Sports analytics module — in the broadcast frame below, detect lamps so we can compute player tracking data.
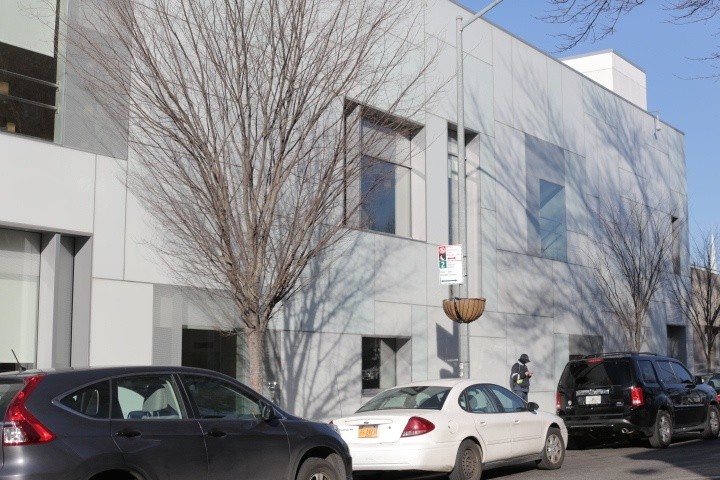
[650,111,660,130]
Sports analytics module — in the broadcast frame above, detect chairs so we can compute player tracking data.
[463,395,485,413]
[418,397,438,408]
[77,390,99,416]
[143,384,179,419]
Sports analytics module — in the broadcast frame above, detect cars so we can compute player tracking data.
[698,373,720,401]
[1,365,353,480]
[328,377,569,480]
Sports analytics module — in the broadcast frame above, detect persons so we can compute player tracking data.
[512,354,533,401]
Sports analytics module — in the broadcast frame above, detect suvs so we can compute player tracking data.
[555,349,720,448]
